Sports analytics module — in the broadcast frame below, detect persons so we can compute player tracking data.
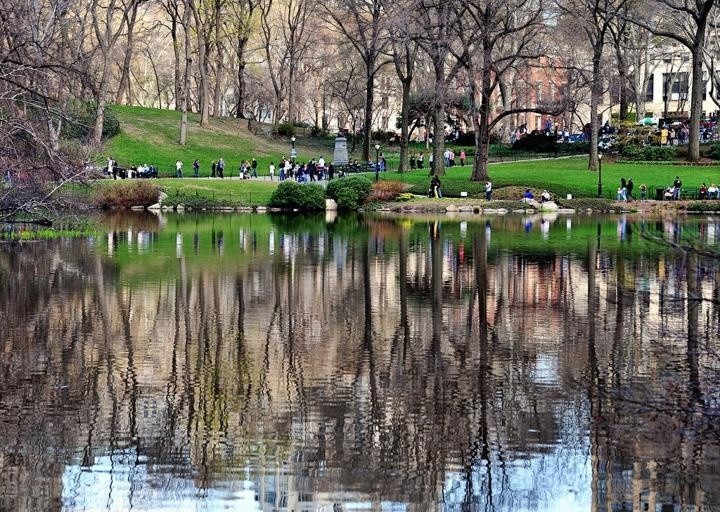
[524,189,535,198]
[698,181,720,199]
[444,147,457,167]
[239,157,257,180]
[5,221,30,234]
[192,159,199,178]
[210,157,225,178]
[672,176,682,201]
[540,189,551,202]
[347,153,387,173]
[418,152,424,169]
[4,160,29,184]
[617,176,637,201]
[279,156,334,182]
[175,160,183,178]
[640,184,646,200]
[107,156,157,181]
[427,175,443,199]
[615,213,720,245]
[430,153,433,168]
[597,113,719,147]
[460,149,465,166]
[485,179,493,201]
[269,162,275,180]
[409,153,416,170]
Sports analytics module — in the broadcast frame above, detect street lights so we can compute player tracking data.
[597,152,603,196]
[291,135,296,169]
[374,144,381,180]
